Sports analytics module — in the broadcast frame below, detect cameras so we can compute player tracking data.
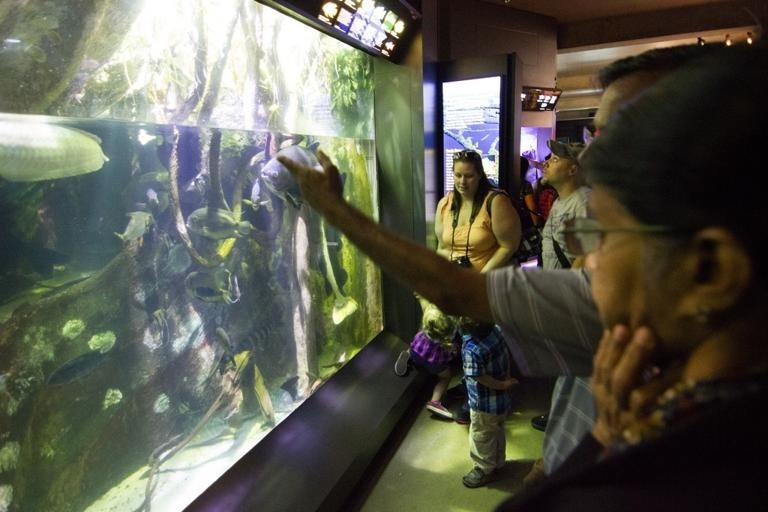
[451,255,472,269]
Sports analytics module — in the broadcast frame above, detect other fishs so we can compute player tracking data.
[1,110,364,431]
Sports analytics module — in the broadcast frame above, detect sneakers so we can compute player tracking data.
[461,462,508,489]
[425,399,454,420]
[393,349,411,376]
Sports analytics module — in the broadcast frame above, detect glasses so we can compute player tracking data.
[451,150,479,162]
[555,215,673,257]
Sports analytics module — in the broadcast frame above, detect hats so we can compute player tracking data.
[545,139,585,162]
